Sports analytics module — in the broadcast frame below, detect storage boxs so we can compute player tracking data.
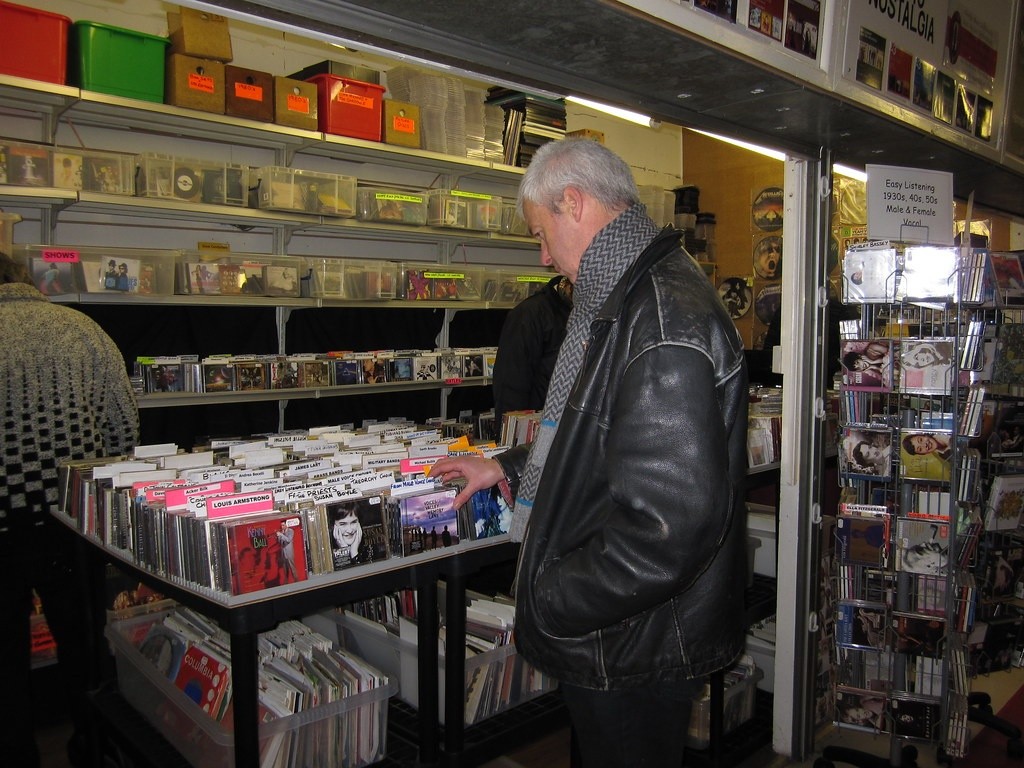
[223,64,275,124]
[0,138,558,307]
[638,181,717,270]
[686,666,765,753]
[73,20,172,103]
[272,74,319,132]
[302,581,566,730]
[164,5,234,61]
[381,97,423,149]
[166,53,225,114]
[105,597,401,767]
[284,60,387,142]
[0,1,72,85]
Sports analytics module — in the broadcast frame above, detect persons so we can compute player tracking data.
[275,520,300,584]
[721,281,749,318]
[105,259,130,291]
[0,253,141,767]
[832,248,1020,741]
[758,238,784,277]
[491,273,585,447]
[329,502,367,567]
[464,356,484,376]
[363,360,385,384]
[423,139,754,768]
[191,265,207,294]
[411,525,452,550]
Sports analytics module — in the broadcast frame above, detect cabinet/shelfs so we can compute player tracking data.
[0,70,563,410]
[45,503,578,767]
[826,222,1022,743]
